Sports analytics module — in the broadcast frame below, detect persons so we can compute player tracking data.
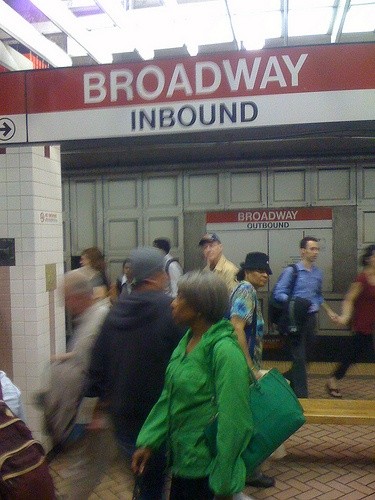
[0,233,375,500]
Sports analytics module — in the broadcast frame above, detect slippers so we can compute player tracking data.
[326,383,342,398]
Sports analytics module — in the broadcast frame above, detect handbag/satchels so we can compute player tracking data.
[268,293,313,336]
[204,367,307,482]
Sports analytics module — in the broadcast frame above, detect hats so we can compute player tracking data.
[128,246,166,279]
[199,233,220,246]
[240,252,272,275]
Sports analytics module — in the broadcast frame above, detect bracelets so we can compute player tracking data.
[329,314,336,320]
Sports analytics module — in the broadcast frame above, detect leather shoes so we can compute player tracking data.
[245,476,275,488]
